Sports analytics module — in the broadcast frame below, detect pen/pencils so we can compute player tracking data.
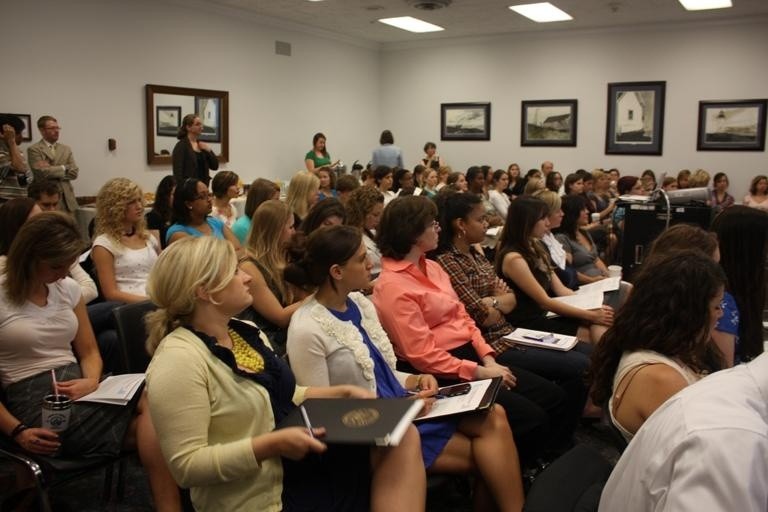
[51,369,59,402]
[522,335,544,342]
[404,390,444,399]
[299,405,315,438]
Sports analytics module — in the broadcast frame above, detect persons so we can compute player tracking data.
[369,128,402,177]
[0,162,768,512]
[419,141,445,171]
[171,113,220,191]
[0,111,35,205]
[303,133,341,177]
[26,114,79,210]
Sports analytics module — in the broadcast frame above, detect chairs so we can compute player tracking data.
[524,445,612,511]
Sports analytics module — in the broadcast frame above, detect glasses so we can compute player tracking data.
[189,191,213,201]
[42,126,60,131]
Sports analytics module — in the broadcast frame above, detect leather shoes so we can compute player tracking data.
[521,457,554,491]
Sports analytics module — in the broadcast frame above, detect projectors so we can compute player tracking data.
[663,187,712,207]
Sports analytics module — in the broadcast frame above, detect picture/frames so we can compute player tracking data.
[440,101,490,141]
[605,81,665,155]
[157,106,181,136]
[10,114,32,141]
[194,95,220,142]
[697,99,767,151]
[520,99,577,147]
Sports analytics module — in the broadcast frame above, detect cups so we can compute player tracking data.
[608,264,623,279]
[41,395,72,434]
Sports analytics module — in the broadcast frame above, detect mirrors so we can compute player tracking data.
[146,84,228,164]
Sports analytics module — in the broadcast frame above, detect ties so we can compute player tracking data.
[50,145,55,155]
